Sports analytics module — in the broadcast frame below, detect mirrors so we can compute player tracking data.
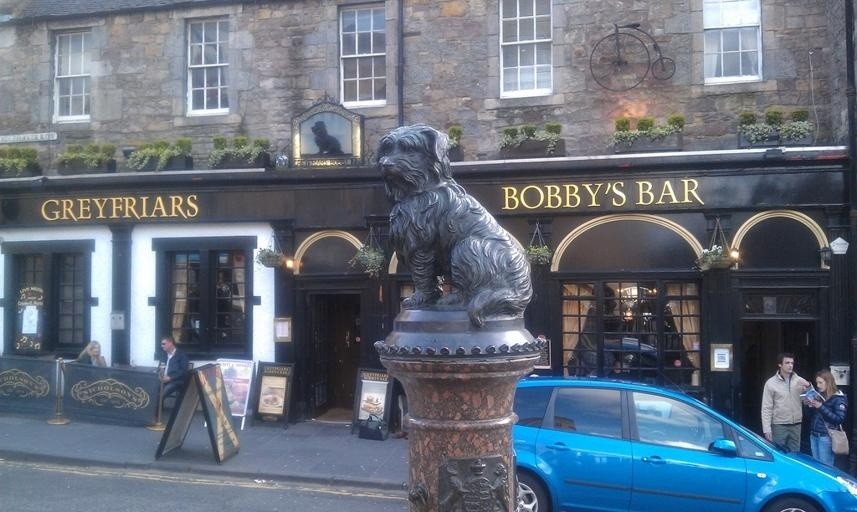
[291,90,364,169]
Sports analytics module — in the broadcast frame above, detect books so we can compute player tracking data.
[800,388,825,409]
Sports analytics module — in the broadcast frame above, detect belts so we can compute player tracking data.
[811,431,826,437]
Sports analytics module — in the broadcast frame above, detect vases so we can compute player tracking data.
[706,257,731,270]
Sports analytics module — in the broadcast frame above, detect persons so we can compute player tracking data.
[763,350,813,453]
[804,368,849,467]
[160,334,188,399]
[78,340,101,365]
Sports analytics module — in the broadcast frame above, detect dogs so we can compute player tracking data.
[377,124,534,329]
[311,120,344,155]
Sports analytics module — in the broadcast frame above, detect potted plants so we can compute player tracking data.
[208,135,270,169]
[0,144,42,177]
[122,137,193,172]
[445,126,464,162]
[524,245,553,265]
[256,246,286,268]
[736,108,814,149]
[496,123,566,159]
[606,112,686,153]
[53,143,116,175]
[348,246,386,276]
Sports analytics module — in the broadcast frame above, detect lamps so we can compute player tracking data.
[285,257,294,270]
[730,244,739,260]
[818,245,832,261]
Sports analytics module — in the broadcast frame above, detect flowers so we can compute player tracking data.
[691,245,738,261]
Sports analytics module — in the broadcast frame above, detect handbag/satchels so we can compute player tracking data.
[827,428,850,456]
[358,414,389,441]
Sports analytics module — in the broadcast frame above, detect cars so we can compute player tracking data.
[511,377,857,512]
[600,339,675,379]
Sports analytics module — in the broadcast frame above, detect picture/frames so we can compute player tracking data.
[710,343,735,372]
[275,317,293,343]
